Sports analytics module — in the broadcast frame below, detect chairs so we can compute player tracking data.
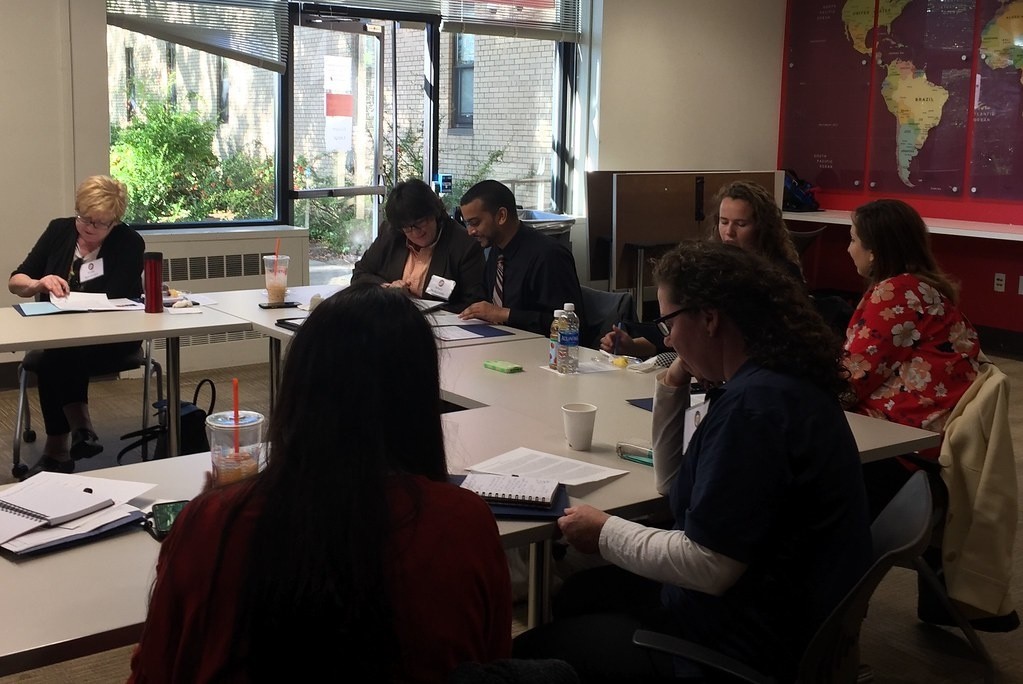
[10,285,1009,684]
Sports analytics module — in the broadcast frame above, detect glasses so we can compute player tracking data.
[398,214,430,233]
[77,214,116,229]
[653,301,698,337]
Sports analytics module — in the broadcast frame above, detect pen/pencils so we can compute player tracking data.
[62,285,65,290]
[613,323,621,355]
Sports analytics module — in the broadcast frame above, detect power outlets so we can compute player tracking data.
[994,273,1005,292]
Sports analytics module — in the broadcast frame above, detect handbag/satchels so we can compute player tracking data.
[152,396,213,461]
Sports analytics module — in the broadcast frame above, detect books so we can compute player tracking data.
[458,472,560,510]
[0,479,115,546]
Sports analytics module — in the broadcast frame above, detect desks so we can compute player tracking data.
[0,406,668,684]
[439,337,940,466]
[188,286,546,426]
[0,305,254,479]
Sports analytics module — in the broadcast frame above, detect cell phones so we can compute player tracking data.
[484,360,523,373]
[258,302,297,309]
[152,500,192,537]
[690,383,706,394]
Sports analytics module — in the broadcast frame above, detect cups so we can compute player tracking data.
[205,411,264,487]
[263,254,290,303]
[562,403,598,451]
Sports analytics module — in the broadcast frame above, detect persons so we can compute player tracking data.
[129,280,515,684]
[601,182,805,363]
[507,236,870,684]
[834,198,982,501]
[456,180,584,345]
[8,175,146,475]
[349,178,488,312]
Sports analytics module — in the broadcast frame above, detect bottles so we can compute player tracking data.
[549,310,569,370]
[558,303,579,374]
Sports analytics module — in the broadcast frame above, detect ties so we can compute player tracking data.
[492,254,504,325]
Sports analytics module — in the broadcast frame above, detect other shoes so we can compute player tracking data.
[69,426,105,461]
[19,453,76,483]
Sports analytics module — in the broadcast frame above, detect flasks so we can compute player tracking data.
[145,251,163,313]
